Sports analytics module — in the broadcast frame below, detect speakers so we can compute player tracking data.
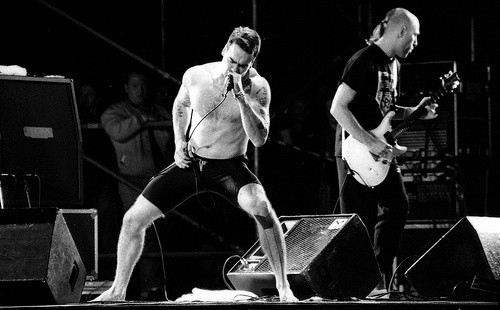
[0,208,87,306]
[404,217,500,299]
[226,213,382,301]
[0,75,87,206]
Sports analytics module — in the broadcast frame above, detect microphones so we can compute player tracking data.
[226,75,233,91]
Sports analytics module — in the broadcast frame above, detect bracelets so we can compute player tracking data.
[234,90,246,100]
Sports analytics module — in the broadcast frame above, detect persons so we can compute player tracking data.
[283,96,340,214]
[87,26,299,303]
[330,7,439,300]
[78,72,171,213]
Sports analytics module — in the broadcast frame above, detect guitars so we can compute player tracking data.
[340,70,463,189]
